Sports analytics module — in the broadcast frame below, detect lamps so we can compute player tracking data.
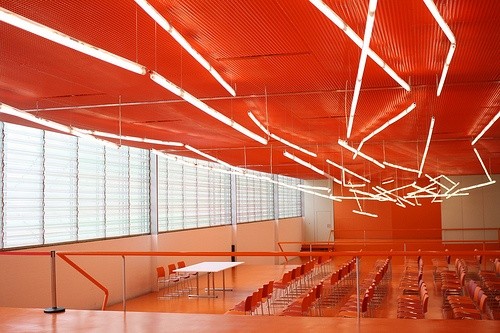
[1,1,499,218]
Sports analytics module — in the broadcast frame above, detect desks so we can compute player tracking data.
[173,261,244,298]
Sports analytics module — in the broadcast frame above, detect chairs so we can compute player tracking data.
[156,266,171,298]
[177,260,192,293]
[225,253,499,320]
[167,264,181,296]
